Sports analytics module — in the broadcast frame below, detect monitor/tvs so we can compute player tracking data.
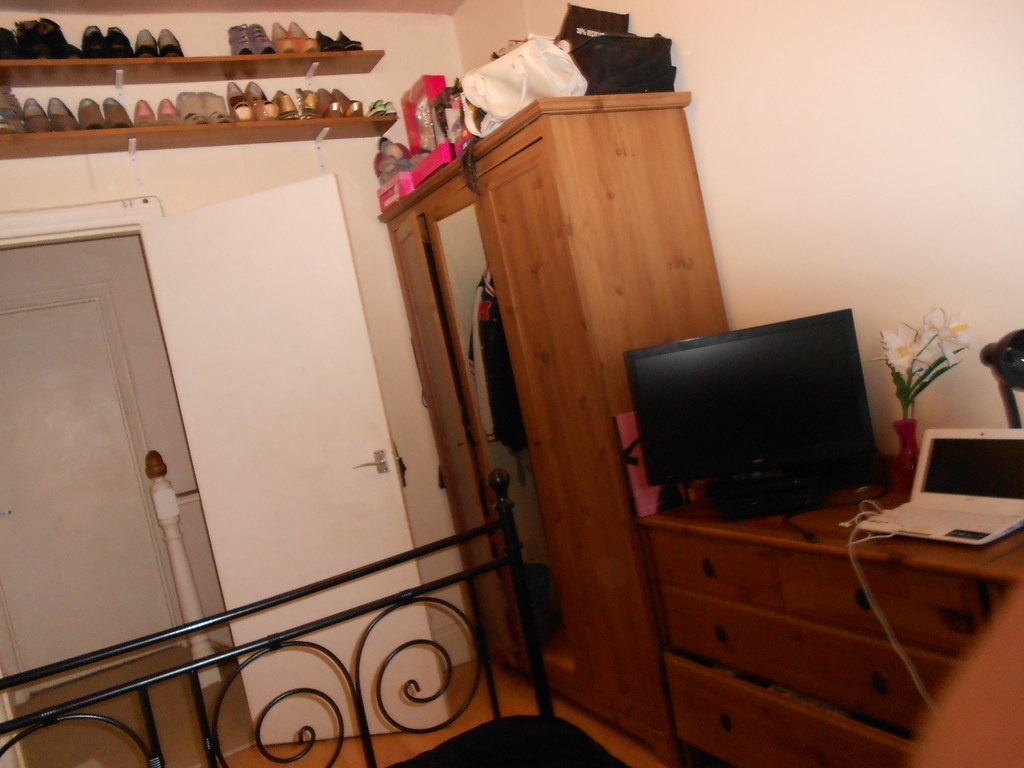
[625,306,876,486]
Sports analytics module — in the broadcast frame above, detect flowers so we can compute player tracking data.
[870,305,970,421]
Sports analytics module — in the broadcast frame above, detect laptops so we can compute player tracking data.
[856,428,1024,546]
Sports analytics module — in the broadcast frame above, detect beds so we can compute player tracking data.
[0,469,631,768]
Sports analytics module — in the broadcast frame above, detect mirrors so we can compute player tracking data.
[435,203,574,669]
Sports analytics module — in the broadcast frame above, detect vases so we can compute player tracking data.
[893,419,921,488]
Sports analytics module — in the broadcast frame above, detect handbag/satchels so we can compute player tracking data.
[461,32,677,136]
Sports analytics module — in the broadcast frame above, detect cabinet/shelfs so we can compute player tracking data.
[379,93,730,768]
[0,50,400,161]
[635,454,1024,768]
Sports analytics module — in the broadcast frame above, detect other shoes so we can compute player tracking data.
[0,83,398,134]
[1,17,364,61]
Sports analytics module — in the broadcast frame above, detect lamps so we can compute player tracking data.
[979,329,1024,429]
